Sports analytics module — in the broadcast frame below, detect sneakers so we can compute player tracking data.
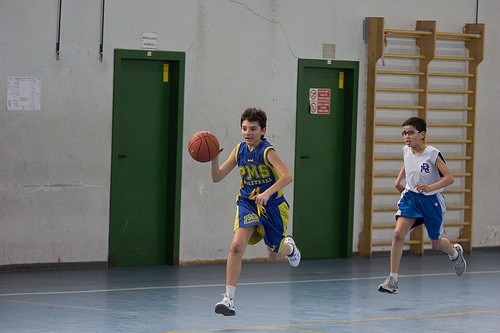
[214,293,236,316]
[377,275,399,294]
[284,235,302,268]
[449,243,466,277]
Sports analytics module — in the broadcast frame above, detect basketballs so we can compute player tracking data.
[187,131,219,163]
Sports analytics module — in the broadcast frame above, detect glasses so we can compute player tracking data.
[401,130,423,137]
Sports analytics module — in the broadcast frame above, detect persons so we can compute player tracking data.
[212,109,301,316]
[377,116,467,294]
